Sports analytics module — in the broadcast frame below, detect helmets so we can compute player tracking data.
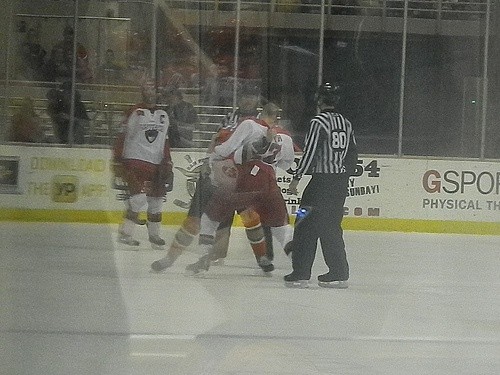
[316,82,343,99]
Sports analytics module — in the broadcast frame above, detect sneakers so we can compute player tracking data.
[117,230,140,251]
[257,254,274,277]
[149,234,166,251]
[151,256,173,273]
[318,271,349,288]
[183,255,211,278]
[284,269,311,288]
[208,248,224,267]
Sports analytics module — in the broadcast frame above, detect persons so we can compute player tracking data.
[150,84,267,272]
[8,26,199,150]
[283,80,359,288]
[186,101,296,271]
[110,77,175,247]
